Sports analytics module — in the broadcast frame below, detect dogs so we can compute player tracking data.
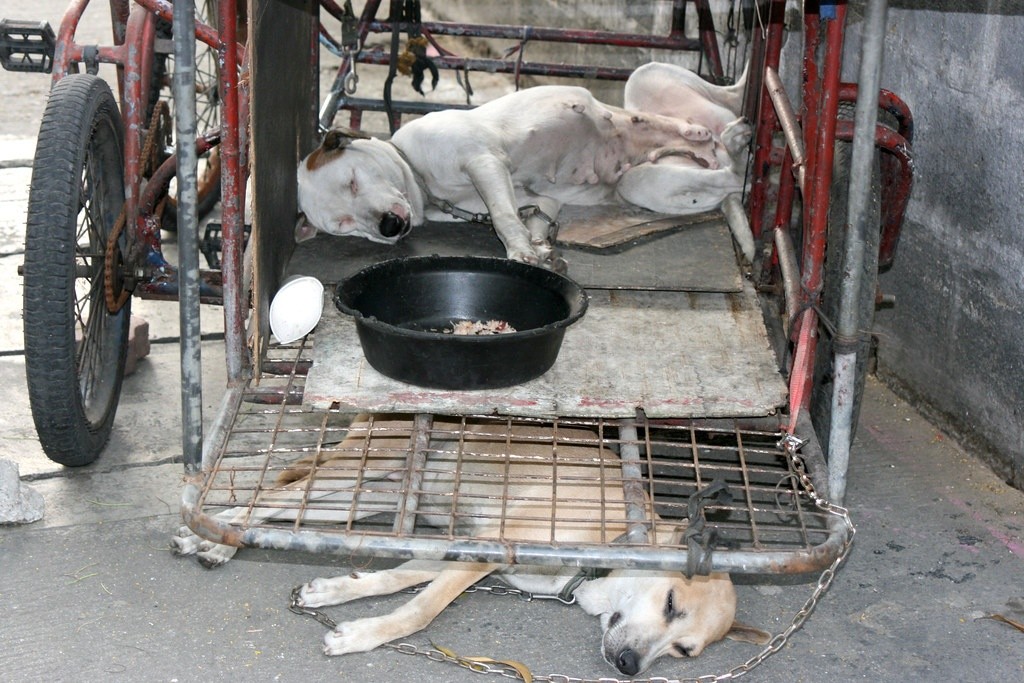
[295,58,759,265]
[169,414,773,677]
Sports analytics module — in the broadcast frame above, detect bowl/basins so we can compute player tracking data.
[333,254,590,391]
